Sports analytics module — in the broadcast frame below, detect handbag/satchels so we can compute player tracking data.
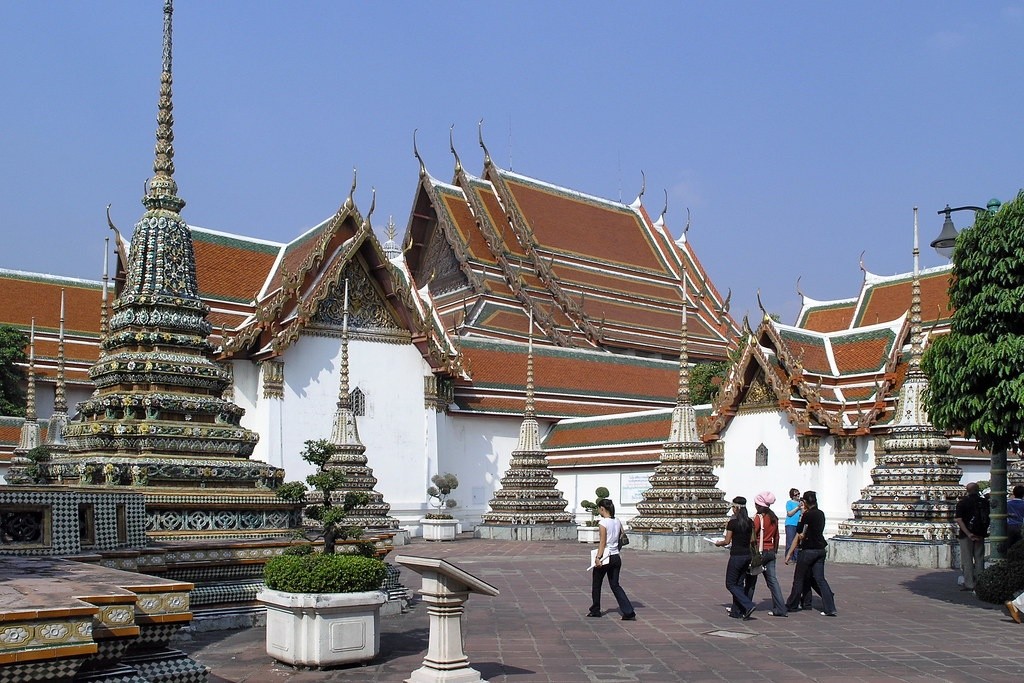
[618,528,629,550]
[969,517,990,538]
[749,554,765,576]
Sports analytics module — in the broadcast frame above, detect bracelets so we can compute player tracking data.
[596,556,601,559]
[798,505,801,507]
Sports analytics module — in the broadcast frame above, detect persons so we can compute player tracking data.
[715,495,757,620]
[1007,485,1024,528]
[956,482,990,592]
[1005,591,1024,624]
[784,492,838,615]
[783,489,802,564]
[725,491,788,617]
[586,498,636,620]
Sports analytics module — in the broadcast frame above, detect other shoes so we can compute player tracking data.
[768,611,783,616]
[821,610,837,616]
[799,603,813,610]
[620,612,636,620]
[785,604,797,612]
[959,584,974,591]
[725,607,732,612]
[586,611,601,617]
[743,606,757,621]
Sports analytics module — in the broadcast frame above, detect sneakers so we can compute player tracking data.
[1005,601,1021,624]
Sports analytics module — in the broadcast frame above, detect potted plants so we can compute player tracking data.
[577,520,601,543]
[256,554,390,667]
[419,512,458,541]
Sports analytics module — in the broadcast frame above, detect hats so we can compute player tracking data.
[726,500,747,508]
[755,492,776,507]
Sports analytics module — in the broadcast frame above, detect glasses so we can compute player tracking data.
[794,493,800,496]
